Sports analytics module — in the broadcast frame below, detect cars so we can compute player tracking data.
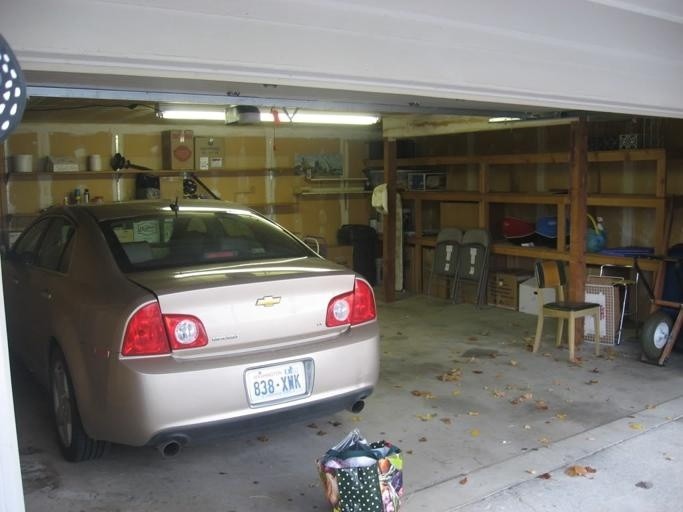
[2,199,381,464]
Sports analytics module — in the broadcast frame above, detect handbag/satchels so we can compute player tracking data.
[316,430,402,512]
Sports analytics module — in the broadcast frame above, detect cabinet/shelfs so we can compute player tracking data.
[1,165,303,218]
[362,148,668,330]
[296,177,374,196]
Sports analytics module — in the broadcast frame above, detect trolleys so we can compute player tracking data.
[630,194,682,370]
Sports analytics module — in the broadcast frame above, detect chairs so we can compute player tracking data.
[120,240,156,265]
[530,259,602,363]
[452,226,490,309]
[599,263,640,345]
[426,226,462,302]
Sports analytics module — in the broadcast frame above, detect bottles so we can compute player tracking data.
[61,188,89,205]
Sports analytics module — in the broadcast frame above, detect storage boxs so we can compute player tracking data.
[194,135,225,168]
[585,274,622,338]
[408,172,448,194]
[161,128,193,168]
[324,245,353,274]
[367,168,418,193]
[463,171,514,194]
[487,268,534,311]
[160,176,185,201]
[440,203,480,232]
[422,248,477,303]
[519,277,556,316]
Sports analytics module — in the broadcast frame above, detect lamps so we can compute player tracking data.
[154,102,383,125]
